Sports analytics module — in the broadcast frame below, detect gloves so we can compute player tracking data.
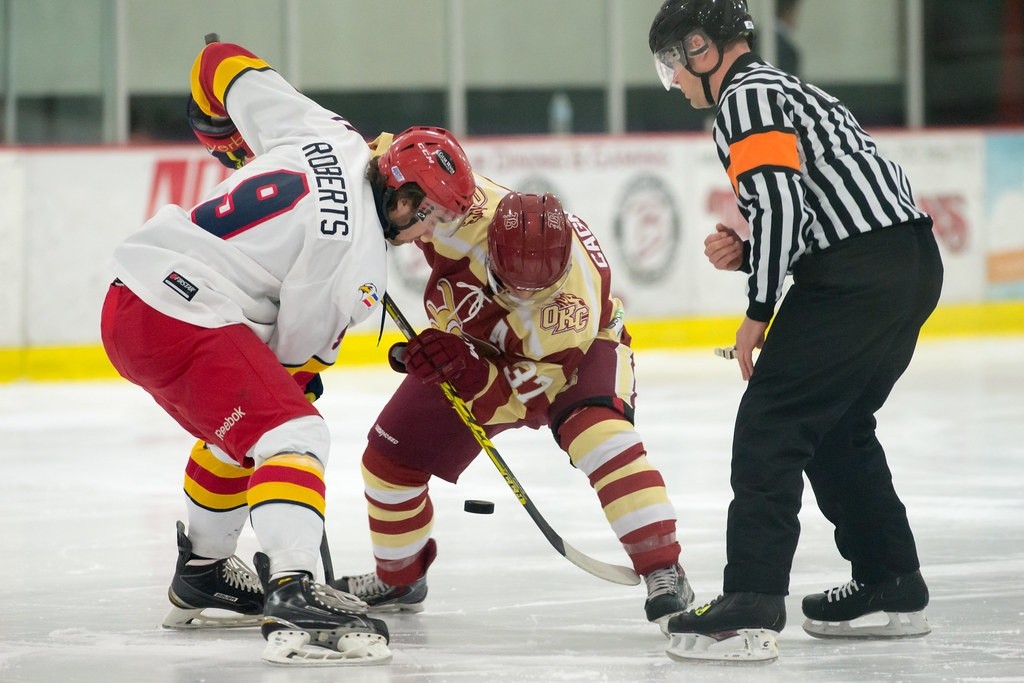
[402,328,490,403]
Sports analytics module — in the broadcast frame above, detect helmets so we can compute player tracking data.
[379,127,477,215]
[649,0,756,76]
[486,189,572,294]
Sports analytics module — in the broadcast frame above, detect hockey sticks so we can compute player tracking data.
[382,293,642,586]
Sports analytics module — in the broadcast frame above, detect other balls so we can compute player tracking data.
[462,498,495,515]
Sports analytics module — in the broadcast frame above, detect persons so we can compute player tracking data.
[335,132,694,633]
[102,44,475,666]
[648,1,944,660]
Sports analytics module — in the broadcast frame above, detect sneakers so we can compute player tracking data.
[801,572,930,641]
[662,594,786,660]
[253,551,394,667]
[326,537,437,614]
[163,519,265,631]
[642,564,696,624]
